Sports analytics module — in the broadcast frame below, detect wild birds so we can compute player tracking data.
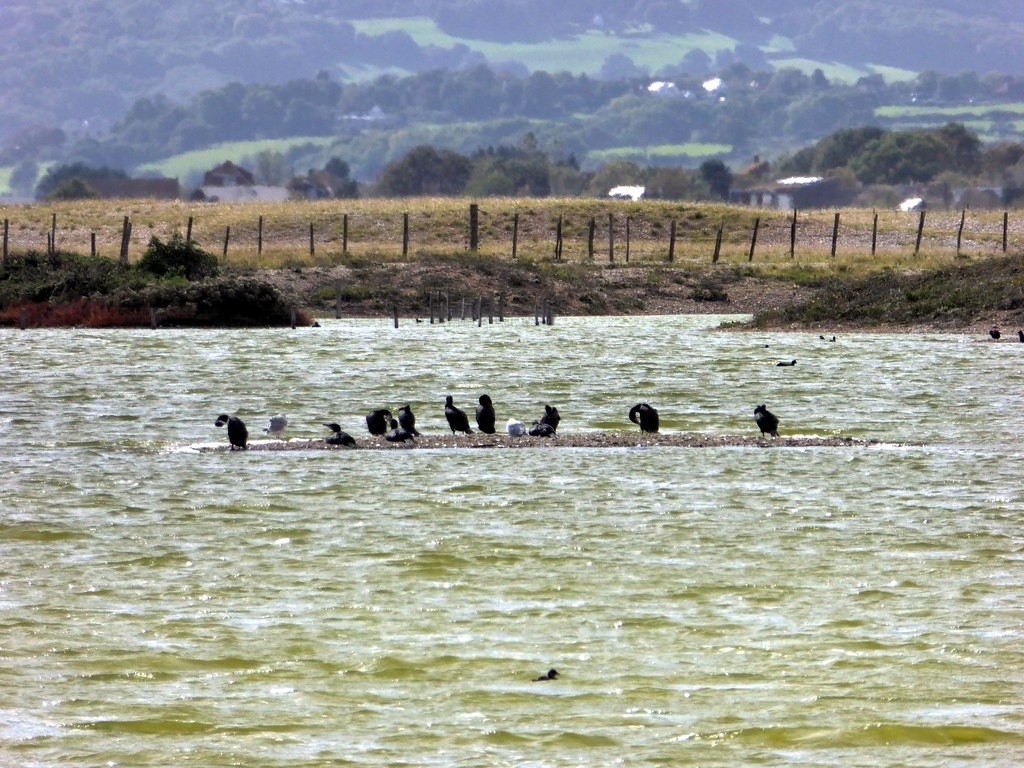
[531,667,562,683]
[775,359,797,367]
[214,412,290,450]
[753,402,783,438]
[629,403,661,436]
[988,329,1024,342]
[415,316,424,323]
[322,405,425,451]
[444,393,561,436]
[764,345,769,348]
[312,321,321,327]
[819,334,837,342]
